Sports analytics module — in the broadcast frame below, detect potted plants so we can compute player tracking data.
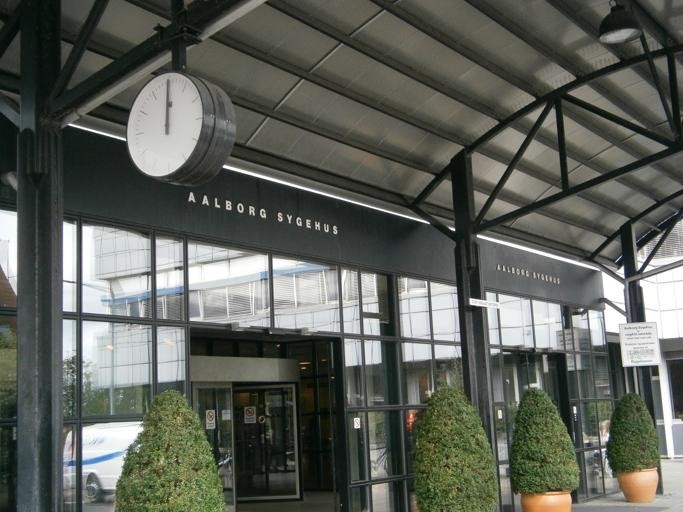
[604,391,660,503]
[507,386,582,512]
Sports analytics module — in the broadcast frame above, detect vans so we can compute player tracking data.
[61,420,147,503]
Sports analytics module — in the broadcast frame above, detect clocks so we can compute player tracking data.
[125,69,237,187]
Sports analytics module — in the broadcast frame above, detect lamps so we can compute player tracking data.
[598,4,643,46]
